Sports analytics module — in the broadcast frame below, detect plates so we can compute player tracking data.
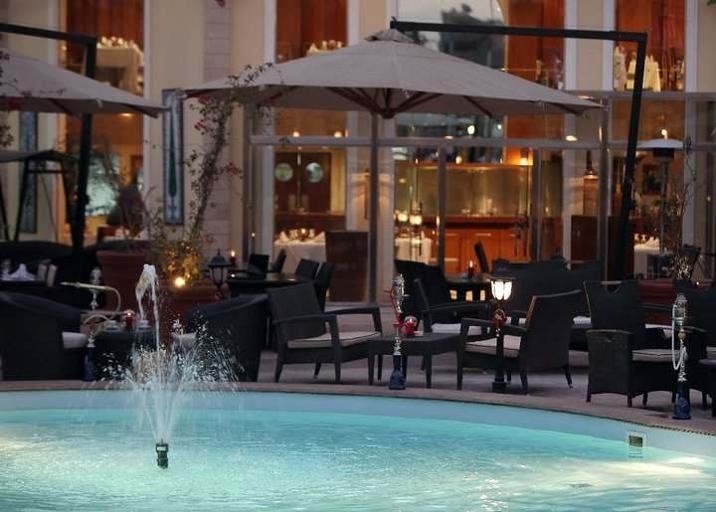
[140,326,153,329]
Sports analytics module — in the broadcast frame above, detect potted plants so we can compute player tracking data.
[88,133,169,310]
[636,136,712,325]
[153,61,286,306]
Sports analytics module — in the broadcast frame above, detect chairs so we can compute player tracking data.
[0,244,716,418]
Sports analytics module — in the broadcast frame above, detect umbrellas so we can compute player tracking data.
[182,20,649,276]
[0,23,169,249]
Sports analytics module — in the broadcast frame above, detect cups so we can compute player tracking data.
[140,320,149,325]
[413,330,424,336]
[505,316,527,324]
